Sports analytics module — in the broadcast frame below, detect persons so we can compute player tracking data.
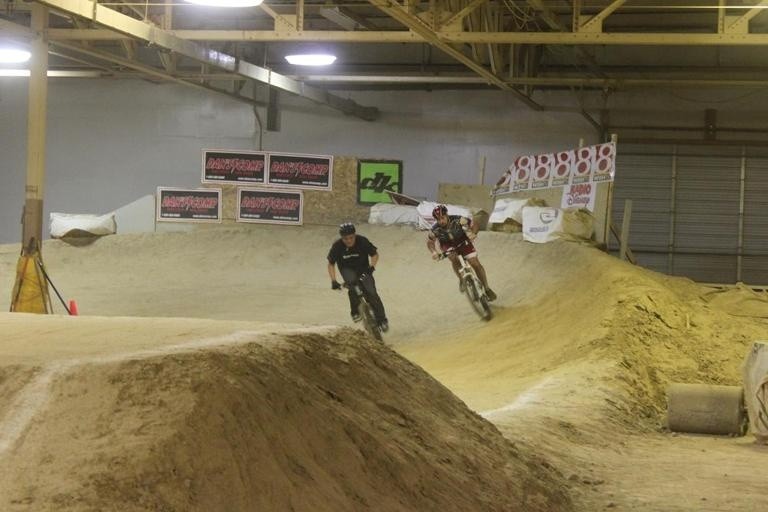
[428,203,497,301]
[327,223,388,332]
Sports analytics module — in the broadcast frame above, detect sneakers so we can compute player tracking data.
[379,321,389,333]
[458,280,468,293]
[486,288,497,300]
[351,311,362,324]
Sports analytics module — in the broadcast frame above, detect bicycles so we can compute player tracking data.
[435,231,494,324]
[336,268,383,344]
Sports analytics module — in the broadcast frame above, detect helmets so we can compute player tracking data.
[337,222,357,237]
[431,204,448,219]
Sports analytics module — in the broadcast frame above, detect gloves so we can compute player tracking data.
[367,266,376,276]
[331,279,342,291]
